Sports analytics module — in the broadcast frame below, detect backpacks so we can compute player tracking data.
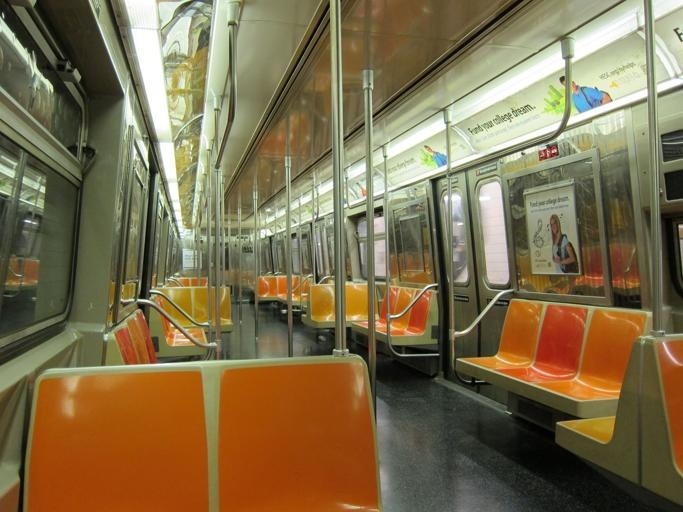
[561,264,577,273]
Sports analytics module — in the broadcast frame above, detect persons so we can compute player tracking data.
[549,215,578,274]
[424,146,448,168]
[559,75,612,113]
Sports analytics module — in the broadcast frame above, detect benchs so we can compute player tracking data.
[518,243,641,296]
[301,283,351,344]
[107,309,158,364]
[554,335,639,506]
[148,287,208,358]
[4,258,38,293]
[165,276,210,287]
[389,252,432,283]
[353,285,441,376]
[23,353,382,512]
[639,331,681,509]
[192,286,231,339]
[456,298,654,425]
[259,275,313,321]
